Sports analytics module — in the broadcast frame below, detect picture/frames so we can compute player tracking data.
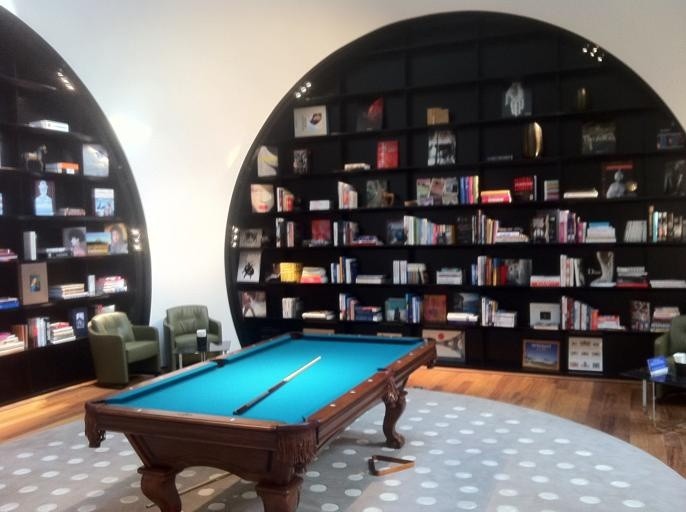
[289,101,331,140]
[420,328,470,365]
[15,259,50,307]
[521,337,560,372]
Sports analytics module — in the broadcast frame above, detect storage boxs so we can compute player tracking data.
[27,119,69,133]
[45,160,78,176]
[309,200,331,210]
[435,269,463,285]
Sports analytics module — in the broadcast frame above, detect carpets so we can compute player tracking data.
[0,387,686,512]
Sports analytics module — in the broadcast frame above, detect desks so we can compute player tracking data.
[84,329,437,512]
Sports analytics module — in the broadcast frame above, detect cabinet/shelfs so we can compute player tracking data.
[224,9,685,383]
[0,8,152,401]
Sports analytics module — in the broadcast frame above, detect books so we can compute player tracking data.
[237,80,686,376]
[0,117,132,358]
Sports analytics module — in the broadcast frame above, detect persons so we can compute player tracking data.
[107,226,127,255]
[241,292,255,319]
[34,181,53,216]
[249,183,274,213]
[67,229,85,257]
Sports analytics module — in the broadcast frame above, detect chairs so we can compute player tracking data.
[655,317,685,402]
[164,304,225,369]
[86,311,164,384]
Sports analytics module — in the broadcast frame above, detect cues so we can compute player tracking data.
[232,355,322,413]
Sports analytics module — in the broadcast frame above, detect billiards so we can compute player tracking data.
[385,391,399,403]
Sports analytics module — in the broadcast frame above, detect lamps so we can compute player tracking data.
[582,43,606,64]
[295,79,312,100]
